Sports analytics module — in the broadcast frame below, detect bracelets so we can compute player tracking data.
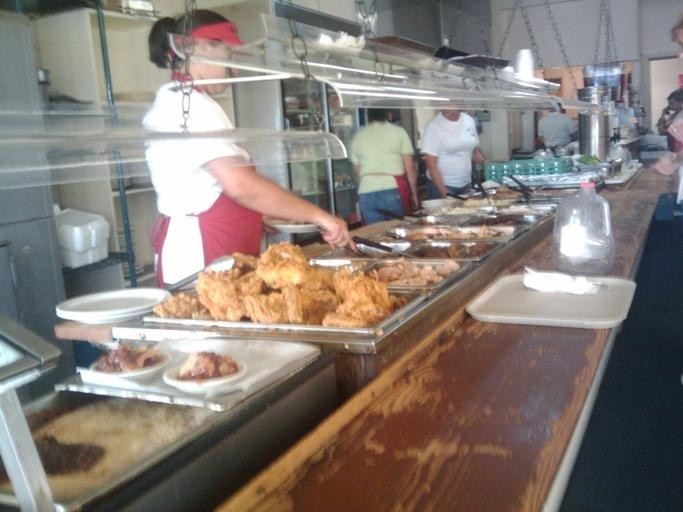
[481,158,487,164]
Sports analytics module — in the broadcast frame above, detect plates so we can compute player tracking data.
[54,285,173,326]
[420,198,447,211]
[263,215,323,234]
[88,355,248,393]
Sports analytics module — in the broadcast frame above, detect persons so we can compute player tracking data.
[658,88,683,217]
[348,108,420,225]
[420,108,488,200]
[536,103,577,155]
[142,9,358,293]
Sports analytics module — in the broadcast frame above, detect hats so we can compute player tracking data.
[174,22,247,48]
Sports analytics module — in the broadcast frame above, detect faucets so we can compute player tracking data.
[608,123,624,143]
